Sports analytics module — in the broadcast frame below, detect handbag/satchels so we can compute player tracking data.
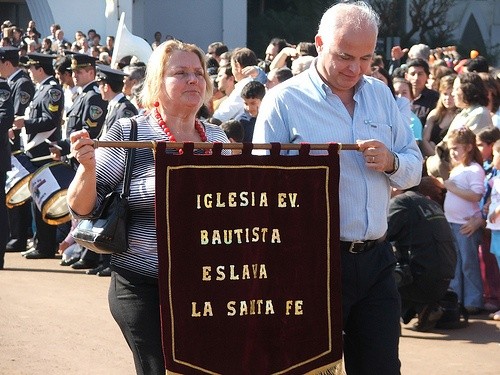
[73,192,131,254]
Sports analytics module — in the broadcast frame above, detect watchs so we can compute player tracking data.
[384,152,399,175]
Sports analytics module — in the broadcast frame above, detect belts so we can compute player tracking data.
[340,231,388,254]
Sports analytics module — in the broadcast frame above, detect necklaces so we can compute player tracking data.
[154,107,212,156]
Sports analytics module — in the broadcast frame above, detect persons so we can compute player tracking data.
[66,38,232,375]
[0,19,500,331]
[251,0,423,375]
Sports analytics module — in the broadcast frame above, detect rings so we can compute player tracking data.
[370,156,375,163]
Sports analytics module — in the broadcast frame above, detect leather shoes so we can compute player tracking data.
[60,258,80,266]
[86,264,112,276]
[21,247,56,259]
[72,259,94,269]
[5,239,26,252]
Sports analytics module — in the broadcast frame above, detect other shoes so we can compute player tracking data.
[412,301,445,332]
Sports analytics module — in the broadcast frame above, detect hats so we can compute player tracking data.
[98,67,129,82]
[0,46,21,59]
[66,53,99,69]
[24,53,58,68]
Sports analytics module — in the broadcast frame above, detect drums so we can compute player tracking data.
[5,151,39,211]
[29,160,78,226]
